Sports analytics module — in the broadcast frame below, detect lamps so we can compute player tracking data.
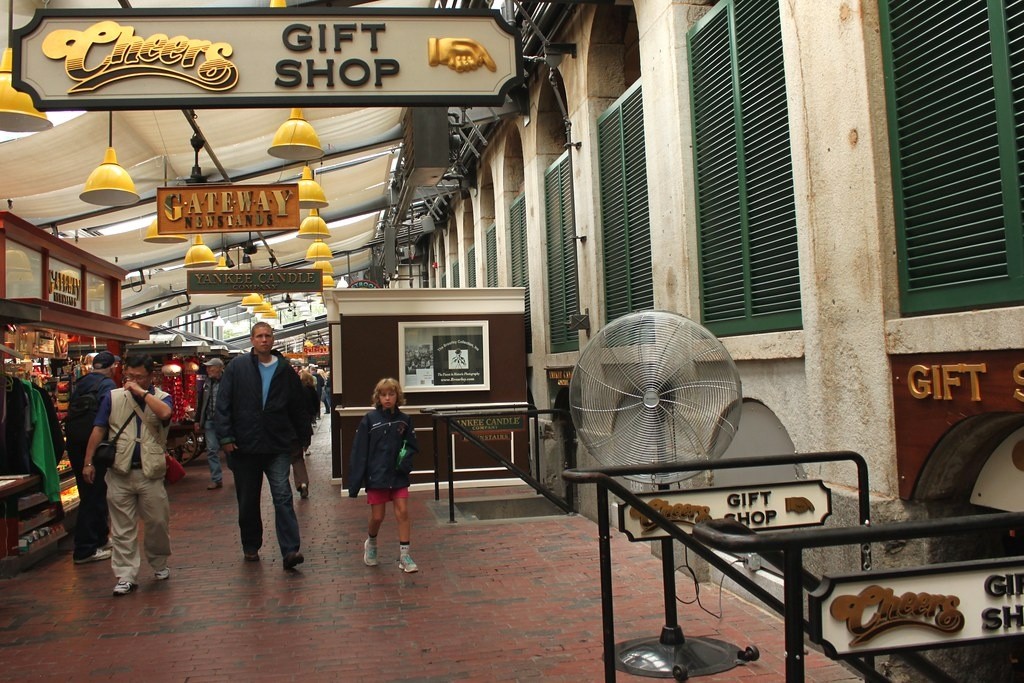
[227,291,279,320]
[182,233,218,267]
[312,262,334,276]
[78,111,142,205]
[266,108,325,162]
[0,33,53,135]
[305,237,335,262]
[143,153,188,244]
[316,275,336,304]
[216,256,229,271]
[298,208,329,238]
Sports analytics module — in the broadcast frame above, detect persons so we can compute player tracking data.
[83,353,172,596]
[214,322,313,571]
[299,371,317,456]
[292,364,332,420]
[65,352,121,564]
[347,378,421,574]
[193,357,225,491]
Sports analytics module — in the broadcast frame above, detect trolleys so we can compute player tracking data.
[166,425,207,467]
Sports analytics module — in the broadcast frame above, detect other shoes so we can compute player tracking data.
[300,483,309,497]
[207,482,223,489]
[284,551,304,569]
[244,551,259,561]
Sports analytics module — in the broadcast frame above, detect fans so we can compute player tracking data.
[281,294,303,319]
[571,311,742,677]
[225,231,262,270]
[267,254,278,271]
[134,127,250,185]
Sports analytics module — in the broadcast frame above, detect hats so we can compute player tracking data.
[92,351,121,369]
[293,361,303,366]
[204,358,225,366]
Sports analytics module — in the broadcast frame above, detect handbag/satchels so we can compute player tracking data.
[94,442,116,467]
[164,452,187,486]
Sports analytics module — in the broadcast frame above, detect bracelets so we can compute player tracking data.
[141,390,150,399]
[84,463,93,466]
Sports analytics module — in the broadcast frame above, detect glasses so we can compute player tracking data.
[125,373,150,381]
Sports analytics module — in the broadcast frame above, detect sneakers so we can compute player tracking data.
[73,549,112,564]
[399,554,419,573]
[113,582,134,594]
[154,566,170,579]
[98,537,113,548]
[364,540,379,567]
